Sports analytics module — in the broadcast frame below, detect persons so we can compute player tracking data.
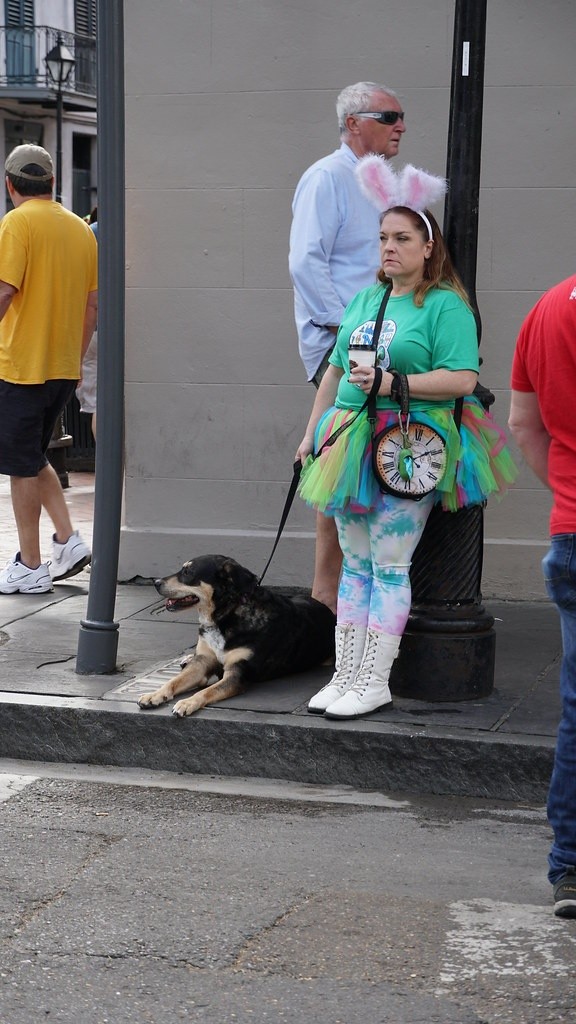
[508,274,576,919]
[0,144,97,594]
[292,205,519,719]
[285,77,407,617]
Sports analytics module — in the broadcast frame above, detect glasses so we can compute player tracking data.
[356,111,404,125]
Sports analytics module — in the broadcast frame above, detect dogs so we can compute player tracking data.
[137,555,337,717]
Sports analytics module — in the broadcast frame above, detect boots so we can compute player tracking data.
[324,626,402,720]
[307,625,367,712]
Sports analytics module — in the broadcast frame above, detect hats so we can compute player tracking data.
[5,144,53,181]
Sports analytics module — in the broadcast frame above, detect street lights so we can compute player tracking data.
[44,31,79,204]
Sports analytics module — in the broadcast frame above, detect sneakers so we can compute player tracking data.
[553,865,576,915]
[49,530,91,581]
[0,551,54,594]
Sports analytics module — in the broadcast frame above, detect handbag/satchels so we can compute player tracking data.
[372,420,448,501]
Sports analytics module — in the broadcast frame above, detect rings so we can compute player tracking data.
[363,376,367,383]
